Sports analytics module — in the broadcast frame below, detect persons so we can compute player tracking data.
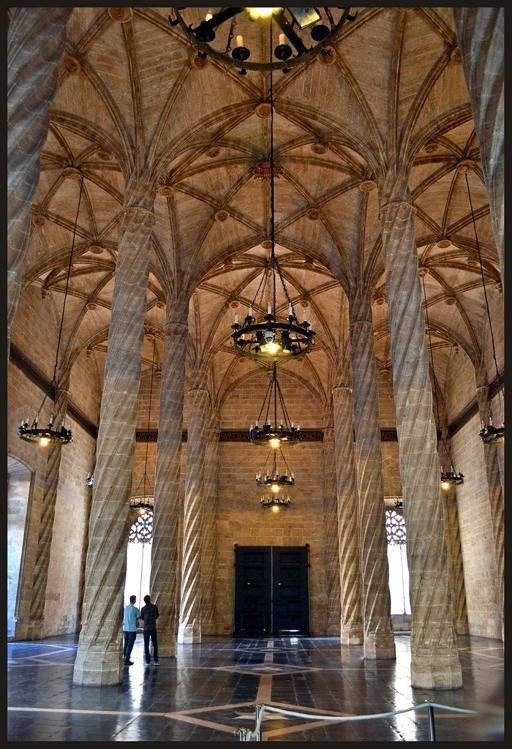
[122,595,142,665]
[140,595,160,666]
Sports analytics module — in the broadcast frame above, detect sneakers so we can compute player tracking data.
[124,660,134,665]
[145,660,151,665]
[153,661,161,665]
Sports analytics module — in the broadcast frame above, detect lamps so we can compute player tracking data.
[131,343,160,515]
[85,472,93,488]
[19,174,91,448]
[421,273,464,489]
[394,490,403,510]
[255,448,295,514]
[229,26,315,363]
[462,170,505,448]
[167,1,360,78]
[249,364,303,450]
